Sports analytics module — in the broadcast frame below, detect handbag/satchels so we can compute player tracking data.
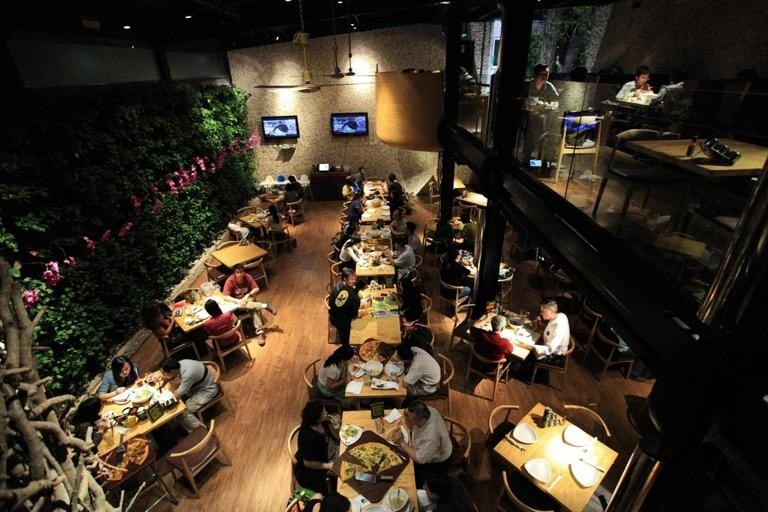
[293,487,316,502]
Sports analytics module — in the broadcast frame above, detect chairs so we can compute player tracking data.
[204,257,227,286]
[176,361,236,427]
[166,419,231,503]
[171,287,252,330]
[216,240,237,249]
[204,320,252,375]
[593,128,664,221]
[534,109,603,183]
[158,334,200,360]
[282,173,660,512]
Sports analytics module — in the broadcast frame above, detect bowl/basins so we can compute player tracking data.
[508,317,525,329]
[640,94,654,103]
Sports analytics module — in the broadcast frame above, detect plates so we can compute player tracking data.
[382,258,391,265]
[512,423,538,443]
[363,205,390,219]
[111,387,174,405]
[563,424,589,446]
[387,487,409,511]
[338,424,364,445]
[359,504,385,512]
[185,306,200,315]
[385,425,409,447]
[569,462,600,488]
[106,418,116,428]
[524,456,552,484]
[347,359,405,378]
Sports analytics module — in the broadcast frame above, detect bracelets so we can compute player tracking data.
[400,441,406,449]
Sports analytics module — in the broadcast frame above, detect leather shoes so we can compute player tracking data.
[257,333,264,345]
[265,303,275,315]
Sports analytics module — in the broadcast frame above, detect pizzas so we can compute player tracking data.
[103,438,149,482]
[358,340,387,363]
[340,441,404,485]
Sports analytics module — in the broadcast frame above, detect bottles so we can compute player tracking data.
[687,134,699,157]
[541,407,552,427]
[495,305,506,316]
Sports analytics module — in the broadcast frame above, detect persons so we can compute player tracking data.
[203,299,241,365]
[431,211,570,386]
[390,399,456,491]
[261,205,284,248]
[282,175,305,222]
[141,302,200,360]
[150,356,220,435]
[645,71,689,111]
[273,121,288,136]
[222,264,277,346]
[314,344,364,409]
[57,397,108,453]
[97,354,142,403]
[342,117,358,133]
[521,63,561,160]
[613,65,662,125]
[300,489,354,511]
[390,344,442,395]
[330,166,434,355]
[291,399,340,491]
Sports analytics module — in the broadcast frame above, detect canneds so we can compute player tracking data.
[540,407,557,428]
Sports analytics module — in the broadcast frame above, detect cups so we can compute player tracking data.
[550,101,558,110]
[116,406,148,428]
[103,433,113,445]
[200,283,210,296]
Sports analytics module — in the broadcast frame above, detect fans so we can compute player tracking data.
[253,27,357,95]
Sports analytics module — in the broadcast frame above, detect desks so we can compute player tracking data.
[311,159,348,201]
[167,286,240,361]
[86,369,187,512]
[627,136,768,233]
[210,241,266,275]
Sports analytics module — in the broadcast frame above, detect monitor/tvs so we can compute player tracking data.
[319,163,330,171]
[262,115,300,139]
[331,112,369,136]
